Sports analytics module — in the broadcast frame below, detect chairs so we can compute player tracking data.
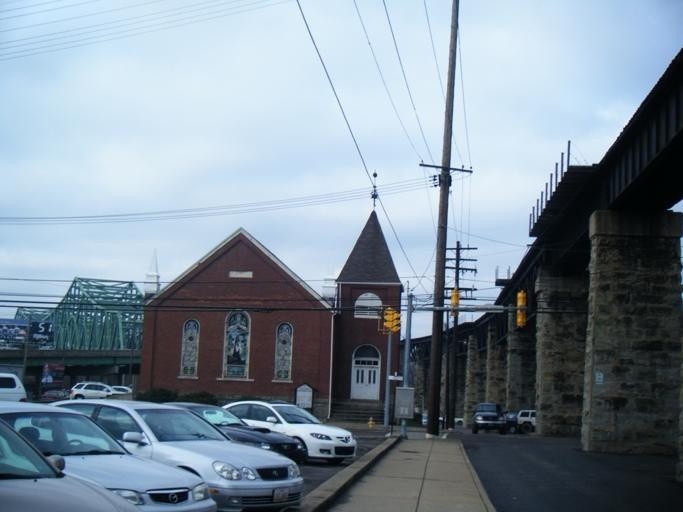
[20,426,42,454]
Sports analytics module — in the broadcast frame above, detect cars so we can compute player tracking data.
[421,410,464,427]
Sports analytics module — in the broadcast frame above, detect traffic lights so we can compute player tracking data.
[383,307,402,333]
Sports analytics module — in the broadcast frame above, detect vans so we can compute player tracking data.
[0,372,28,402]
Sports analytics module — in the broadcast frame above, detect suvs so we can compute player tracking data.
[69,380,132,399]
[471,402,537,434]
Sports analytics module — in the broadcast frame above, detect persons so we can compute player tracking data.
[41,364,53,383]
